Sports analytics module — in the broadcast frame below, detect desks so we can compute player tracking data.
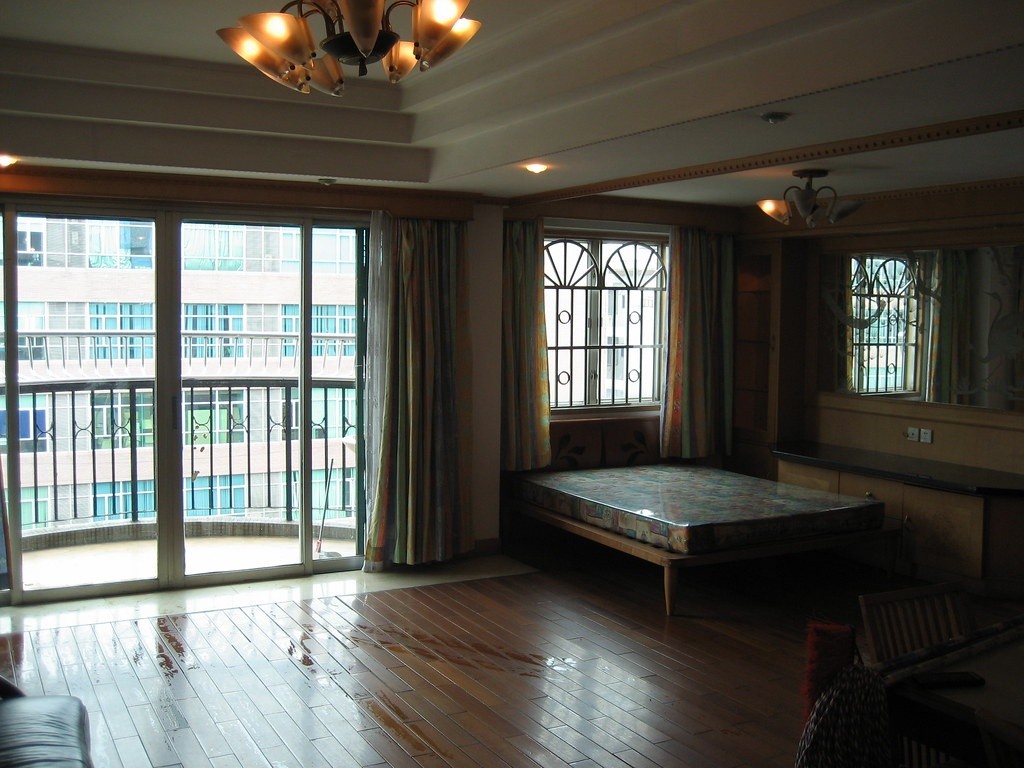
[888,638,1024,768]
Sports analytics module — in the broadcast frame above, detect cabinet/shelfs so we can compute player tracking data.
[766,438,1024,597]
[733,237,806,444]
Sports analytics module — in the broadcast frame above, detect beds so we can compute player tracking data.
[500,416,885,617]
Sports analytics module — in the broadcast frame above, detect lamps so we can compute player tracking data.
[215,0,483,98]
[756,168,863,229]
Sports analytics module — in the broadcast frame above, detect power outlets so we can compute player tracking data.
[920,428,933,444]
[908,427,920,441]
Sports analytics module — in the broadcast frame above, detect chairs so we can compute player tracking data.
[857,580,975,768]
[0,675,94,768]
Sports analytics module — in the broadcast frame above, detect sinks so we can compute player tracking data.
[342,434,356,452]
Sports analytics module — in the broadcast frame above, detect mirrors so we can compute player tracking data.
[802,226,1024,433]
